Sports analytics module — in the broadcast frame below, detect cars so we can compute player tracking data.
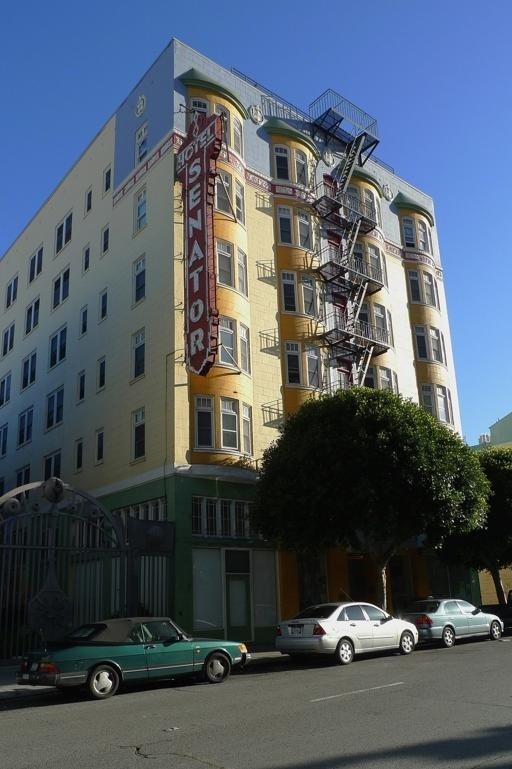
[397,598,505,648]
[15,615,251,700]
[274,601,422,666]
